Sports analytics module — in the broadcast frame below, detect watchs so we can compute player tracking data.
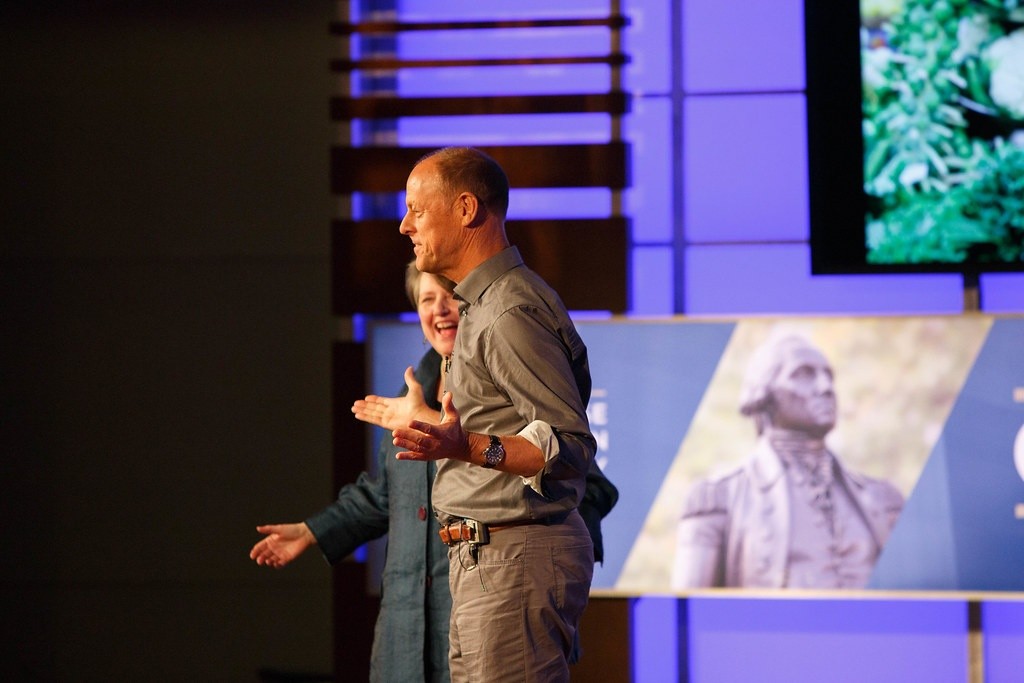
[480,434,504,469]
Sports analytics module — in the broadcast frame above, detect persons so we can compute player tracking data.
[350,146,598,682]
[249,260,618,683]
[672,336,905,590]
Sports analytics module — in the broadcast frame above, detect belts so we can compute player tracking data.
[437,518,541,544]
